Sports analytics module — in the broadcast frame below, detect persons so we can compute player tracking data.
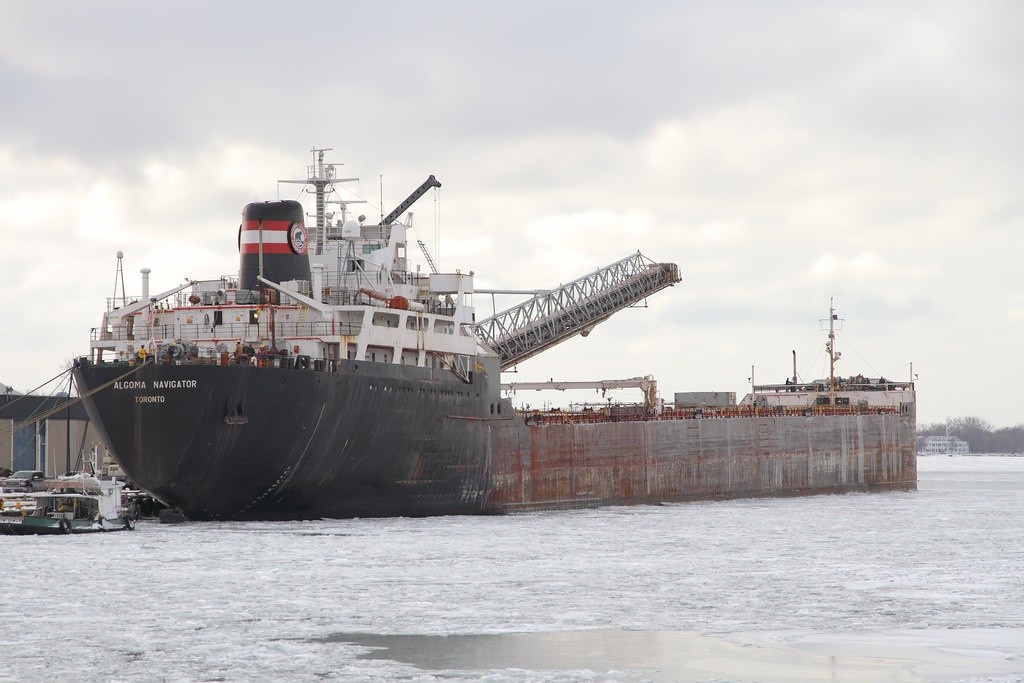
[235,340,267,368]
[138,344,147,364]
[786,377,791,392]
[878,376,885,391]
[166,343,176,364]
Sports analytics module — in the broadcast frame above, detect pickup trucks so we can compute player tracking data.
[3,470,55,493]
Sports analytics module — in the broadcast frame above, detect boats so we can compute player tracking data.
[0,447,138,535]
[71,144,917,522]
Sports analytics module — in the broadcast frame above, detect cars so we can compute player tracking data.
[58,470,101,484]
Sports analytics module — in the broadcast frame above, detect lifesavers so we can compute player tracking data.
[60,517,72,534]
[66,497,72,505]
[125,515,135,531]
[296,354,310,372]
[204,314,210,325]
